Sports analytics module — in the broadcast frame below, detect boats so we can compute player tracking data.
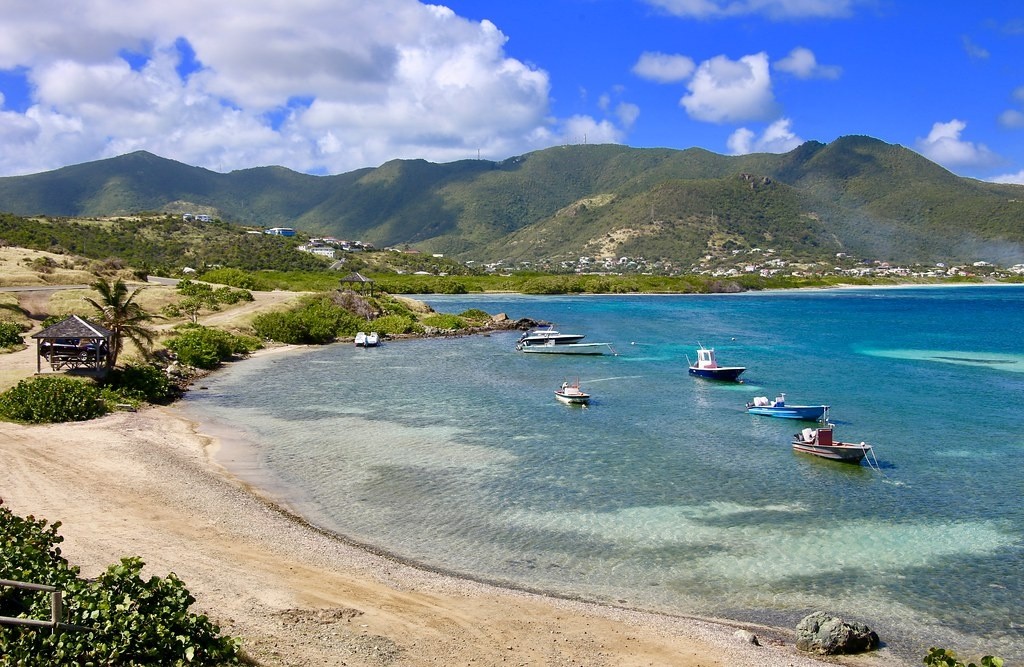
[521,330,585,345]
[745,392,831,421]
[555,381,591,405]
[688,349,746,381]
[791,427,873,465]
[516,339,616,356]
[355,330,379,347]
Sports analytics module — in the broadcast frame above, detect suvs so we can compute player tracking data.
[40,338,105,361]
